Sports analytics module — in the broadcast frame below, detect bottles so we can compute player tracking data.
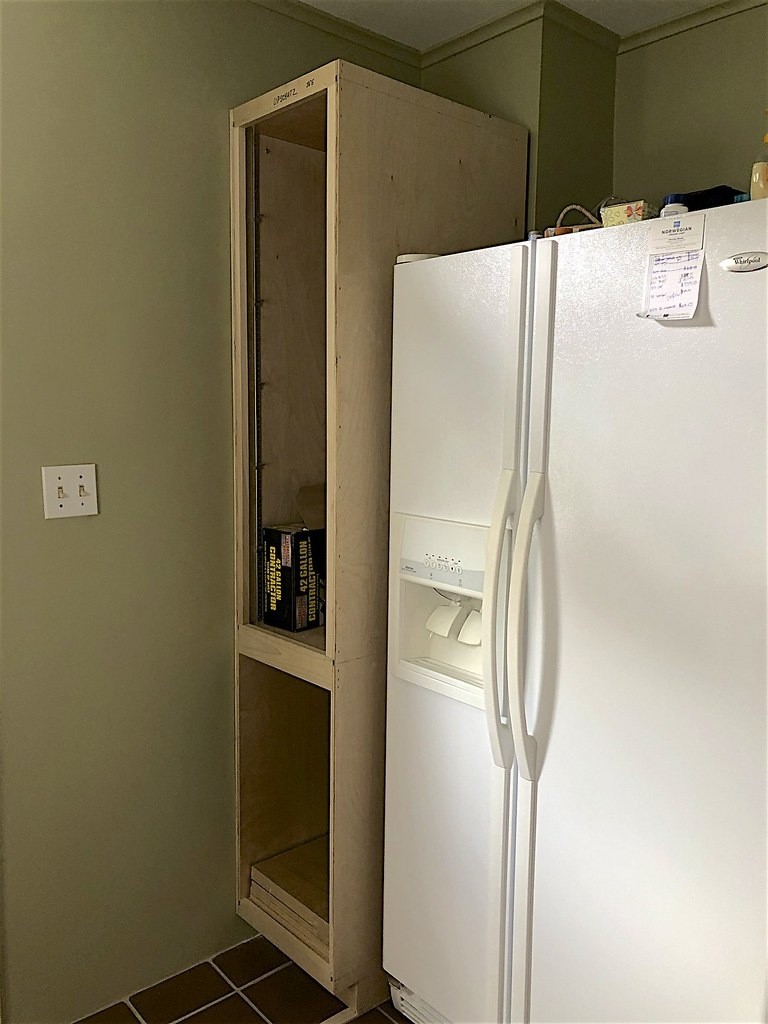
[659,193,688,217]
[749,132,768,200]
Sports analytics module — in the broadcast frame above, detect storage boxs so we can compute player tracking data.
[257,523,326,632]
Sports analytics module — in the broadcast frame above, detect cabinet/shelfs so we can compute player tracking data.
[227,60,530,1016]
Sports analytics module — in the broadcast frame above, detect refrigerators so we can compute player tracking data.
[381,197,768,1024]
